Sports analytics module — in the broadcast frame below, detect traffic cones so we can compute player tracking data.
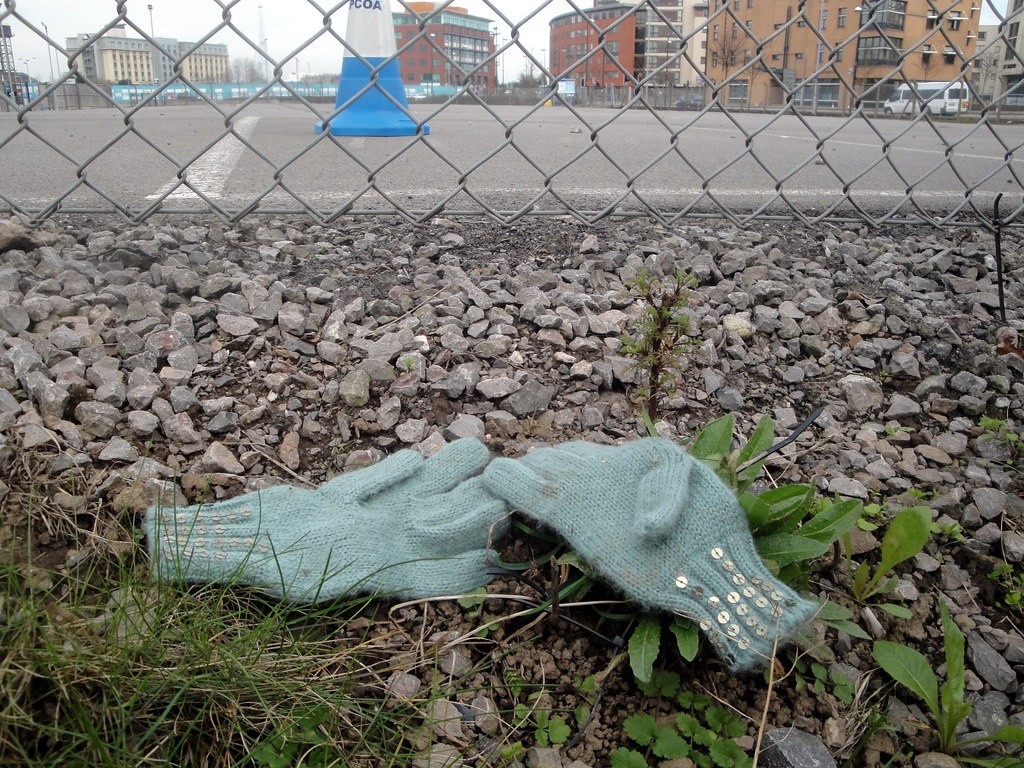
[315,0,431,137]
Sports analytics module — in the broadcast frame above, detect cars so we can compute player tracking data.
[675,95,703,108]
[177,93,201,101]
[407,93,430,101]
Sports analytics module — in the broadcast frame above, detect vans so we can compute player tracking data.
[884,81,969,117]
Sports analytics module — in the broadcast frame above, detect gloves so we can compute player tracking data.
[482,436,814,673]
[148,435,513,602]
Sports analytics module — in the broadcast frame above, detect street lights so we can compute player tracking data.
[541,49,545,87]
[849,6,863,113]
[148,5,154,37]
[295,58,299,81]
[430,33,435,94]
[703,25,708,75]
[494,27,497,78]
[502,39,507,83]
[19,58,36,83]
[264,38,268,78]
[42,22,54,81]
[666,34,672,81]
[586,19,594,86]
